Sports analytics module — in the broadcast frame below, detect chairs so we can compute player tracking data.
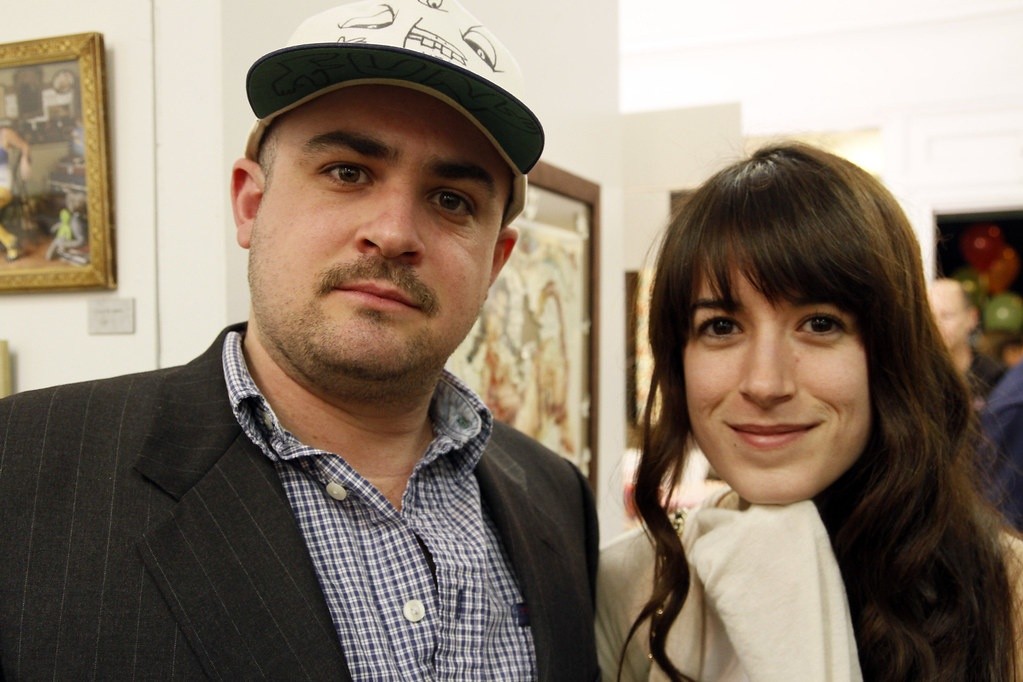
[0,117,45,255]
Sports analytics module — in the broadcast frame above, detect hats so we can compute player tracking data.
[245,0,546,229]
[986,293,1023,332]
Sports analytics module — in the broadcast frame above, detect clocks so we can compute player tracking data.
[51,68,76,95]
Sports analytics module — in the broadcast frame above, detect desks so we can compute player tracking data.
[46,162,91,268]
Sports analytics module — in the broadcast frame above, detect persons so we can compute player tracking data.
[593,139,1023,682]
[0,1,600,682]
[0,126,31,260]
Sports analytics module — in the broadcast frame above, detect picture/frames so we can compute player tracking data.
[0,30,118,297]
[441,156,602,514]
